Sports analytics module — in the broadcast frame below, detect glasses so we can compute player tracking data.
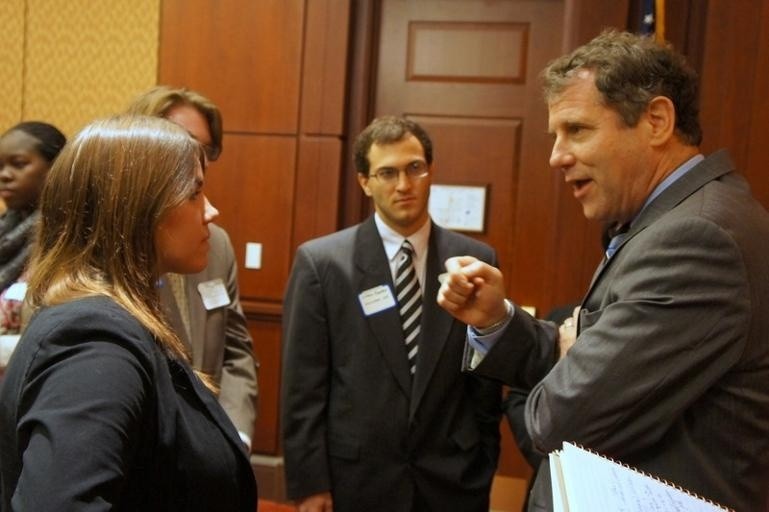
[369,161,428,183]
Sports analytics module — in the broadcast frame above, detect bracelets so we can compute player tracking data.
[472,305,513,335]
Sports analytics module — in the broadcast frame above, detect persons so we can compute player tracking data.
[436,26,768,512]
[0,122,66,335]
[0,113,259,512]
[501,219,619,512]
[276,113,507,510]
[123,84,261,459]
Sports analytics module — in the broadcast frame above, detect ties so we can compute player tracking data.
[394,240,422,381]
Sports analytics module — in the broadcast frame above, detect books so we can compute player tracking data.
[546,451,568,512]
[559,440,735,512]
